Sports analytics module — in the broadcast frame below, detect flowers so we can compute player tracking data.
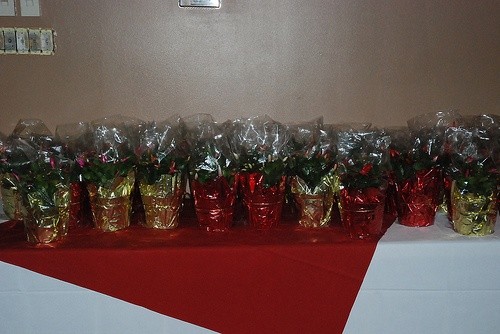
[0,137,500,183]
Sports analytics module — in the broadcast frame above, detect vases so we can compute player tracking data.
[0,175,82,246]
[240,172,286,230]
[138,171,185,230]
[189,172,241,232]
[389,172,439,228]
[444,166,500,236]
[337,186,386,239]
[288,175,337,228]
[87,168,134,231]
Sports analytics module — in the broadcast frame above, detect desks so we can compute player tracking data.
[0,205,500,334]
